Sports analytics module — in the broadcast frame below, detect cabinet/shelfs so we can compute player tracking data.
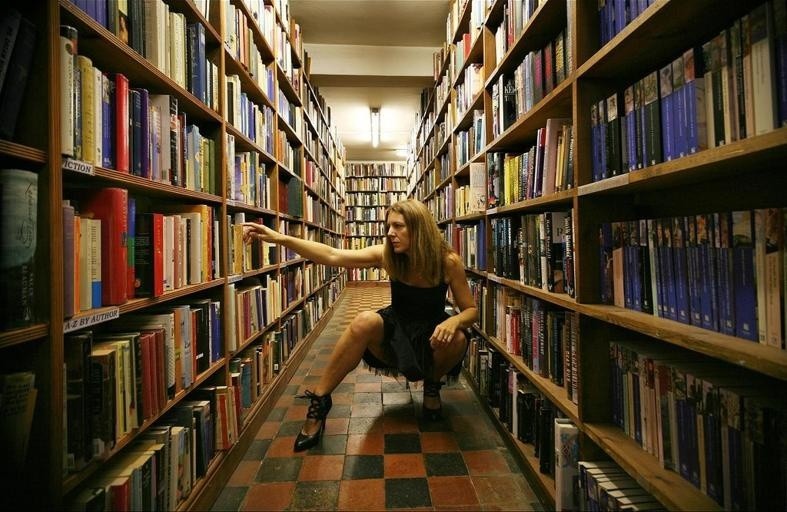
[345,161,406,282]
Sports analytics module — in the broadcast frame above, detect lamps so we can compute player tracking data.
[370,105,380,149]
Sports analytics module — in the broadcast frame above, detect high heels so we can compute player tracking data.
[423,381,445,423]
[293,390,332,451]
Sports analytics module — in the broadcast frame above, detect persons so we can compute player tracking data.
[240,199,479,451]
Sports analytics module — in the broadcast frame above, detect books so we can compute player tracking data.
[1,0,308,510]
[344,164,408,281]
[403,85,431,215]
[306,81,346,331]
[576,3,786,512]
[432,1,574,512]
